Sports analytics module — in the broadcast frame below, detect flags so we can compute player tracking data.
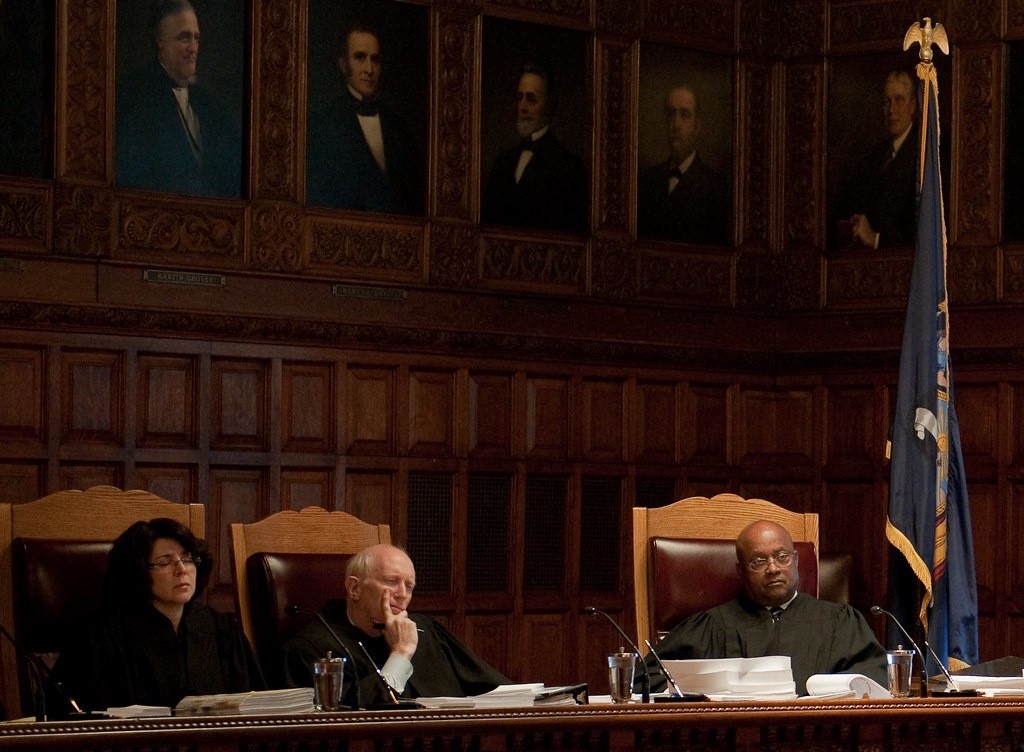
[885,62,981,674]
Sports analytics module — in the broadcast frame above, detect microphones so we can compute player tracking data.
[284,605,360,711]
[871,605,929,698]
[584,606,651,704]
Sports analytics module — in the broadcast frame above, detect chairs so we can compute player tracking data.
[226,505,392,674]
[0,485,206,721]
[632,493,821,661]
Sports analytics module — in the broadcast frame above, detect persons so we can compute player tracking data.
[634,520,890,698]
[272,544,516,709]
[38,517,261,721]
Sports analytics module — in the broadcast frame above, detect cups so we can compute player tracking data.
[886,645,916,698]
[608,647,635,705]
[314,650,347,712]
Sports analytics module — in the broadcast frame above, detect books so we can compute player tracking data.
[175,688,315,716]
[107,704,171,718]
[416,682,577,708]
[945,675,1024,698]
[797,674,893,699]
[588,693,670,705]
[657,656,797,702]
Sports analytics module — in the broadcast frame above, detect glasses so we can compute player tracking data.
[742,553,794,573]
[149,555,202,570]
